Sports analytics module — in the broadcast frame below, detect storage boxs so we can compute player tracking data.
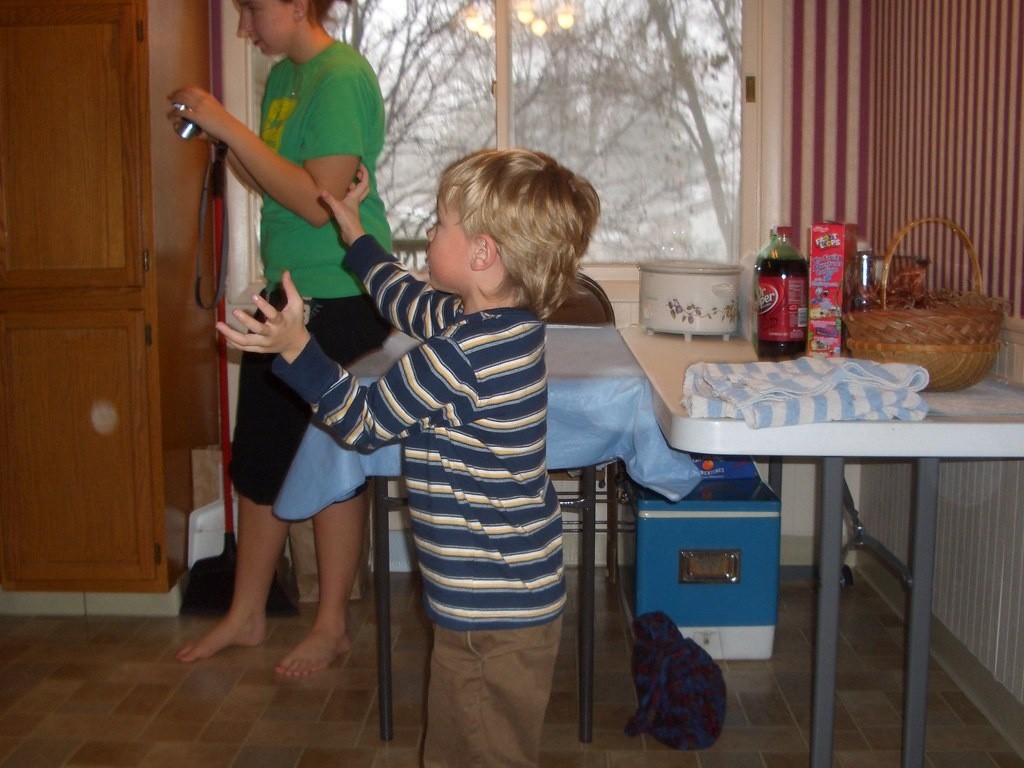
[618,462,781,661]
[807,220,859,360]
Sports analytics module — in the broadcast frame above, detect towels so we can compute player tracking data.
[682,351,934,430]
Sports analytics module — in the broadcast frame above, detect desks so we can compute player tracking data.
[616,326,1024,768]
[271,323,701,742]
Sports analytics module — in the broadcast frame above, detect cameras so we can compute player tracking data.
[173,103,201,140]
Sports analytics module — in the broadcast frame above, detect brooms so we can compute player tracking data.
[176,153,300,620]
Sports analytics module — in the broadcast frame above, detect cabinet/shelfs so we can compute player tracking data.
[0,0,221,620]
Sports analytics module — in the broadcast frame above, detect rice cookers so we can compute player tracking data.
[638,258,744,341]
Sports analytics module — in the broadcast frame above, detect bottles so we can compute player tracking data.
[752,227,778,354]
[757,227,808,360]
[854,243,874,310]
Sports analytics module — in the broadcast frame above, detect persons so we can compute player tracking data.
[214,145,602,767]
[167,1,394,676]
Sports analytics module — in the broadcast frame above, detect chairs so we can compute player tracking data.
[543,271,623,587]
